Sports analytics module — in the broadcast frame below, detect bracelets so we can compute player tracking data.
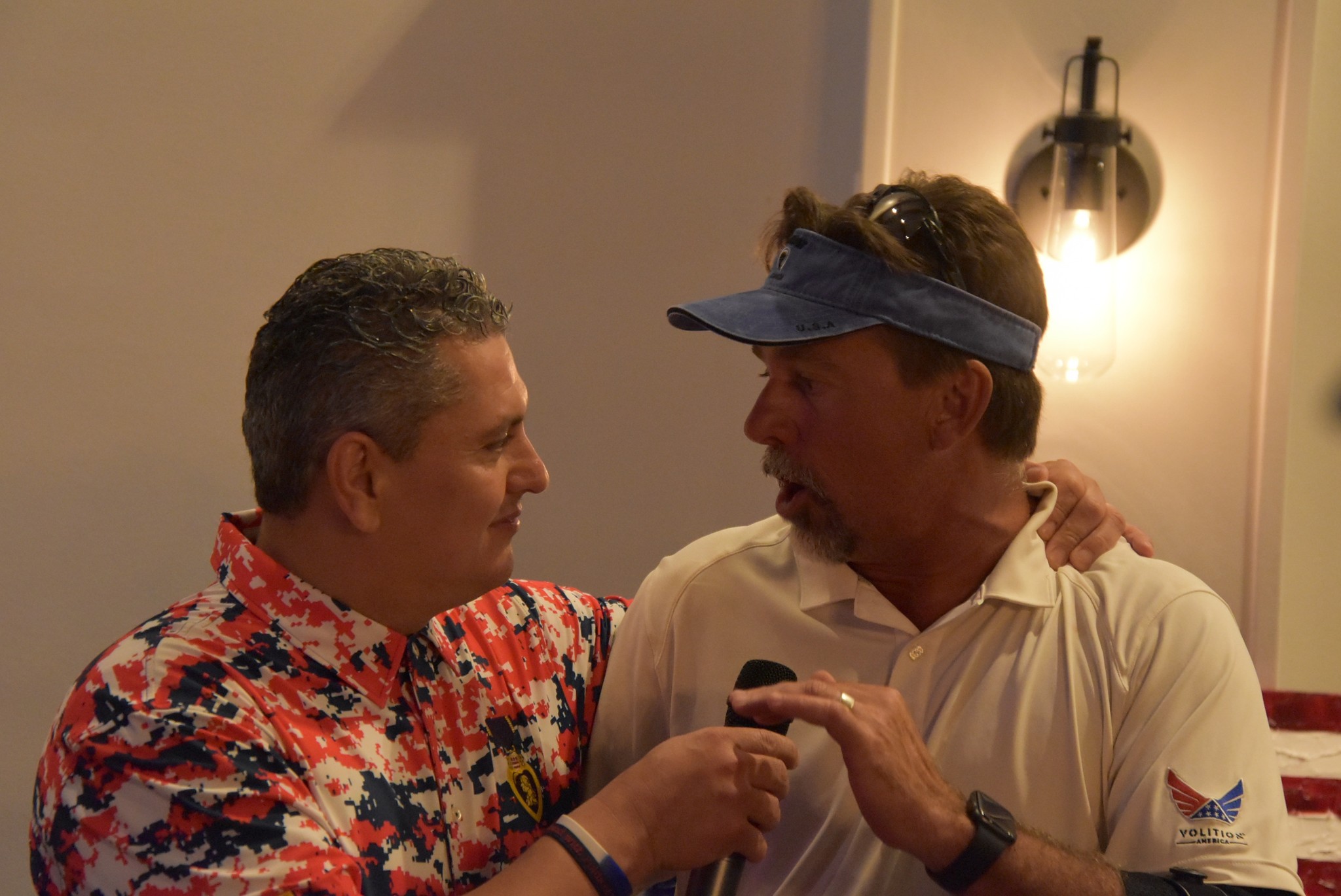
[546,814,633,896]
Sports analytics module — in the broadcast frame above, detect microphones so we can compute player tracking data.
[684,659,797,896]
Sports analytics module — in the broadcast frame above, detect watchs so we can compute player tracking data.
[924,790,1017,893]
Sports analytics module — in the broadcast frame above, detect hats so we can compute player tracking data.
[667,232,1043,373]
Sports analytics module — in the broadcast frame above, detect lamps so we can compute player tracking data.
[1008,36,1150,387]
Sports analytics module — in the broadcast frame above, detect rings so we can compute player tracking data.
[839,692,854,709]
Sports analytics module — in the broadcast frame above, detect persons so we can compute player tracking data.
[581,174,1303,896]
[28,239,1153,896]
